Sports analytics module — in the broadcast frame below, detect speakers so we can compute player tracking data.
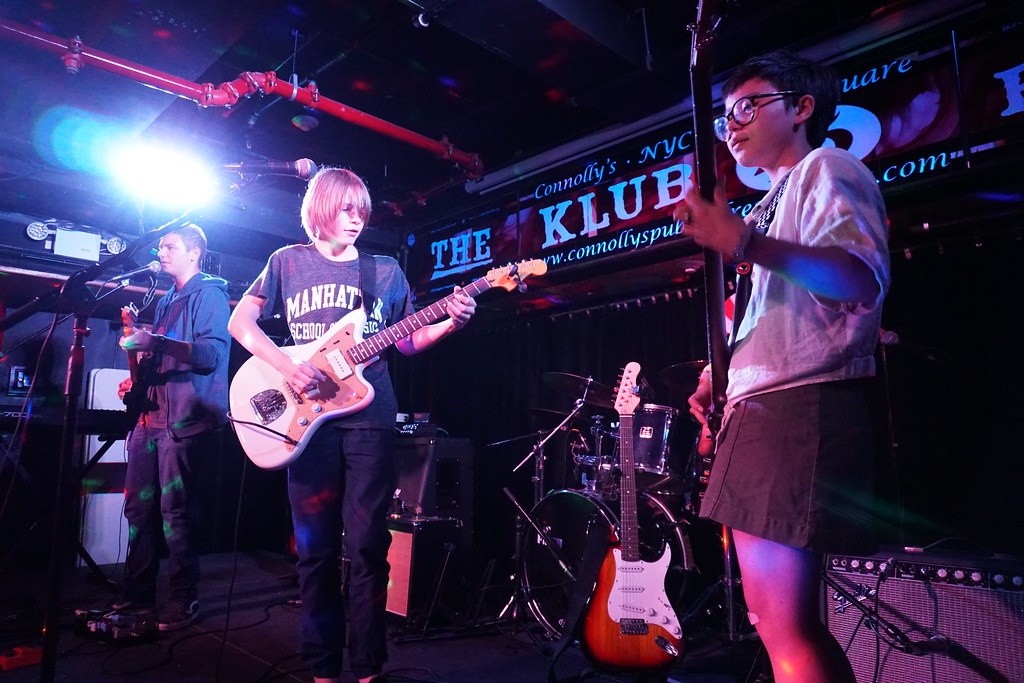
[820,536,1024,682]
[381,513,459,628]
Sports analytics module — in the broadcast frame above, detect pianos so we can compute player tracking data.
[0,403,130,589]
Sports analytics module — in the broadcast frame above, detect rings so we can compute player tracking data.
[685,211,692,224]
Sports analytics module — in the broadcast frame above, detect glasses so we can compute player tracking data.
[713,91,797,142]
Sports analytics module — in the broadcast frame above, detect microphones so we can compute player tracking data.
[562,566,579,582]
[901,635,949,657]
[244,159,317,181]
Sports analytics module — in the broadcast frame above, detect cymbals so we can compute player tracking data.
[531,369,638,413]
[649,359,708,396]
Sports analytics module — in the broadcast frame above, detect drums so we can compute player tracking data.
[609,399,693,479]
[517,486,687,651]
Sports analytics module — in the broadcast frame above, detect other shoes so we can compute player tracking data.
[158,603,200,632]
[74,605,129,623]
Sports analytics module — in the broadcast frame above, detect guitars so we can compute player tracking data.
[584,359,686,667]
[120,303,150,427]
[229,256,549,471]
[685,1,730,446]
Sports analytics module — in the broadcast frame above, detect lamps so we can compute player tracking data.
[26,221,127,262]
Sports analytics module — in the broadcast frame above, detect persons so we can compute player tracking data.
[97,223,231,628]
[672,49,892,683]
[227,168,477,683]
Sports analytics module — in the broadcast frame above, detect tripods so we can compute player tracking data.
[453,488,577,654]
[681,528,743,645]
